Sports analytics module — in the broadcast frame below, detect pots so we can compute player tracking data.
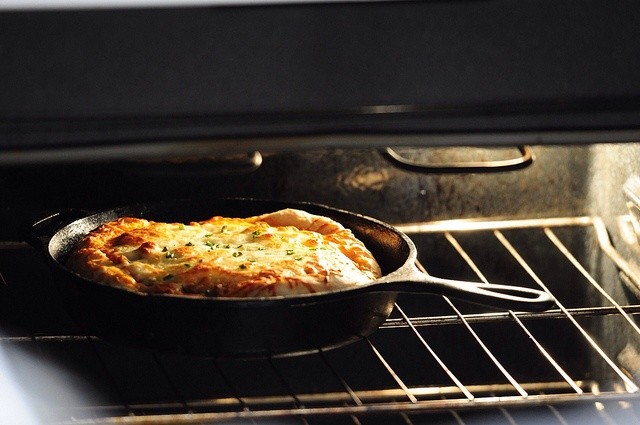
[32,196,553,348]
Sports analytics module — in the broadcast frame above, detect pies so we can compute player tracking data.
[76,207,382,297]
